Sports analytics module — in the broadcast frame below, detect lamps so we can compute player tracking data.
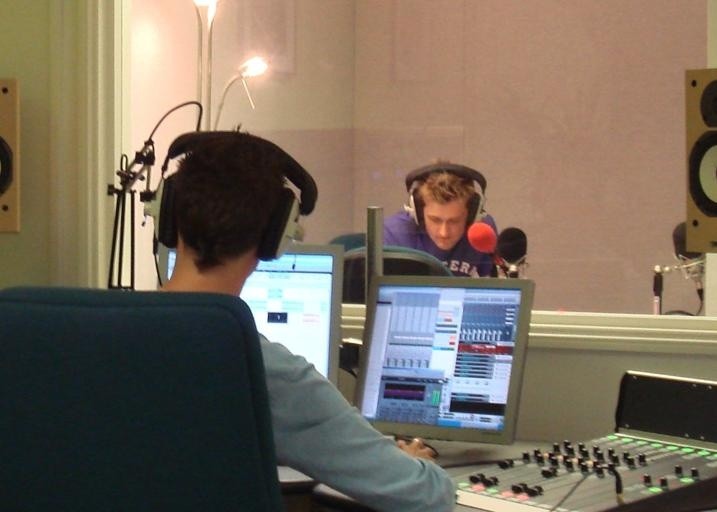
[194,0,268,131]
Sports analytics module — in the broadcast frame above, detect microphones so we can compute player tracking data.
[673,220,704,302]
[467,222,510,278]
[497,226,527,278]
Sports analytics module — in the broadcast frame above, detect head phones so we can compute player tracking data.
[143,129,320,261]
[402,162,487,233]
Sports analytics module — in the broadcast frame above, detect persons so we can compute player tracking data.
[143,131,458,512]
[383,162,499,277]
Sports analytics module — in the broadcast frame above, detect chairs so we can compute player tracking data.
[1,284,285,512]
[344,246,454,304]
[328,232,367,251]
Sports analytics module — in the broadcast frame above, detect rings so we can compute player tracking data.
[413,438,423,444]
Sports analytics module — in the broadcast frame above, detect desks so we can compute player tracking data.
[277,465,315,488]
[313,437,555,512]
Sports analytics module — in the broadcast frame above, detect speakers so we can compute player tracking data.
[686,67,717,253]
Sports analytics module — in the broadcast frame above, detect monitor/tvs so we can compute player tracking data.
[351,274,535,446]
[156,245,343,389]
